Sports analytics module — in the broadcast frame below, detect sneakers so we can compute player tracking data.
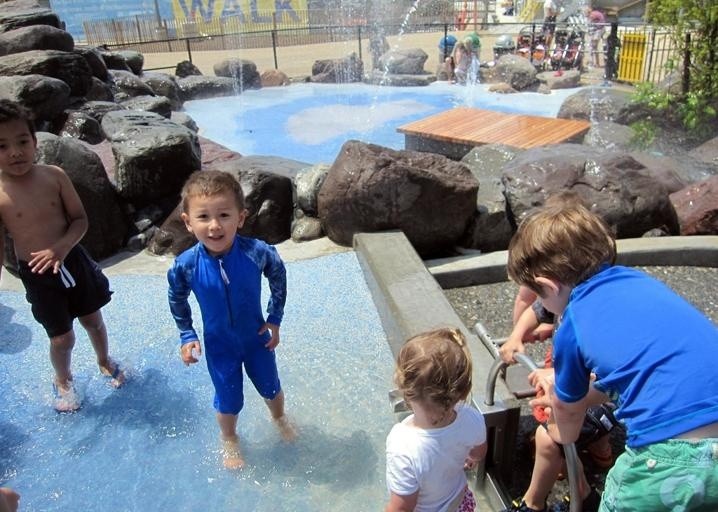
[587,443,613,466]
[499,483,602,512]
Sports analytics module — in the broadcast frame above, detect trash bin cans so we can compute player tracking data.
[493,36,514,60]
[439,34,480,64]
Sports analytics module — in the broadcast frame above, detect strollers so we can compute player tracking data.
[516,26,586,71]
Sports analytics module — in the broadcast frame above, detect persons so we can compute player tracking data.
[388,328,487,511]
[499,202,718,512]
[0,97,125,414]
[445,0,607,86]
[167,170,298,475]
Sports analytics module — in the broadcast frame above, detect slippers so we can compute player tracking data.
[52,381,83,412]
[101,358,131,388]
